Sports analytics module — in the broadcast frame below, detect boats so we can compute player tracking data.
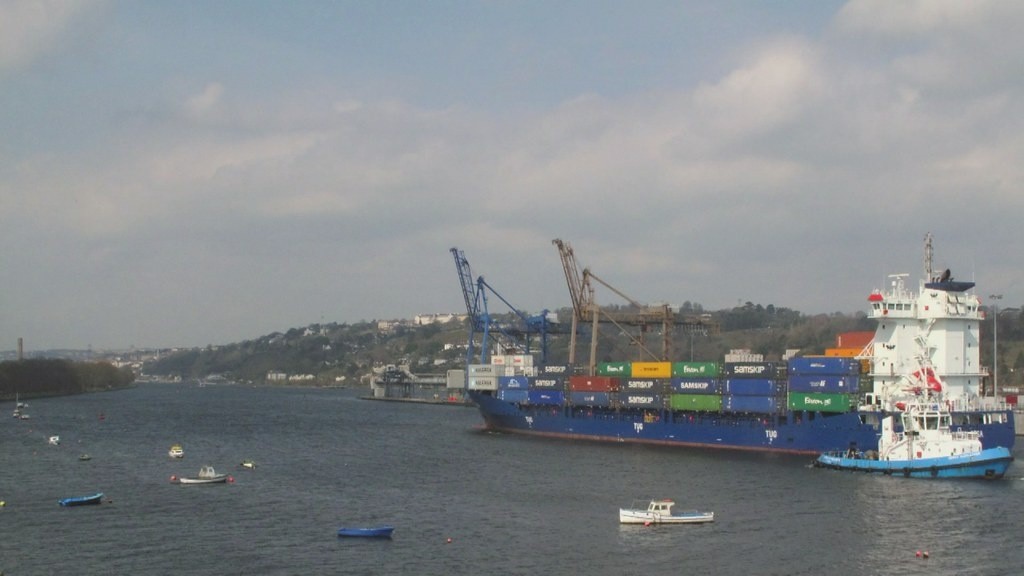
[176,465,231,485]
[813,362,1017,481]
[336,524,396,539]
[55,489,104,507]
[49,435,61,447]
[168,443,185,460]
[79,454,91,461]
[618,497,717,527]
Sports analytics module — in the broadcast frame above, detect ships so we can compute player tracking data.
[450,230,1020,465]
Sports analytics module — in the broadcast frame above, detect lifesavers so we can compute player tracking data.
[663,498,673,502]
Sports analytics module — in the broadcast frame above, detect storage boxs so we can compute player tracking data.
[446,331,877,413]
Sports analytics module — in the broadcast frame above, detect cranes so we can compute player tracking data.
[553,237,722,378]
[450,246,590,368]
